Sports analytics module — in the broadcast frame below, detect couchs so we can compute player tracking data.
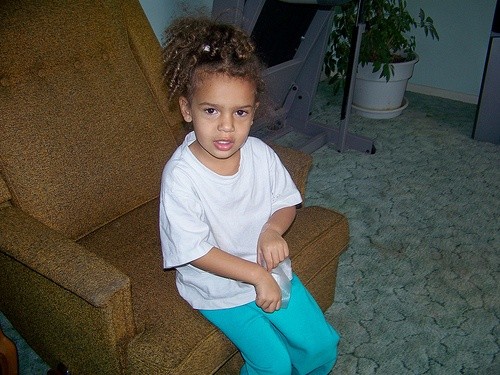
[0,0,351,374]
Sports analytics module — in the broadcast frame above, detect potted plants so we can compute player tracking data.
[320,0,439,120]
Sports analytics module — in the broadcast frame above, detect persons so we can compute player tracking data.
[156,10,341,375]
